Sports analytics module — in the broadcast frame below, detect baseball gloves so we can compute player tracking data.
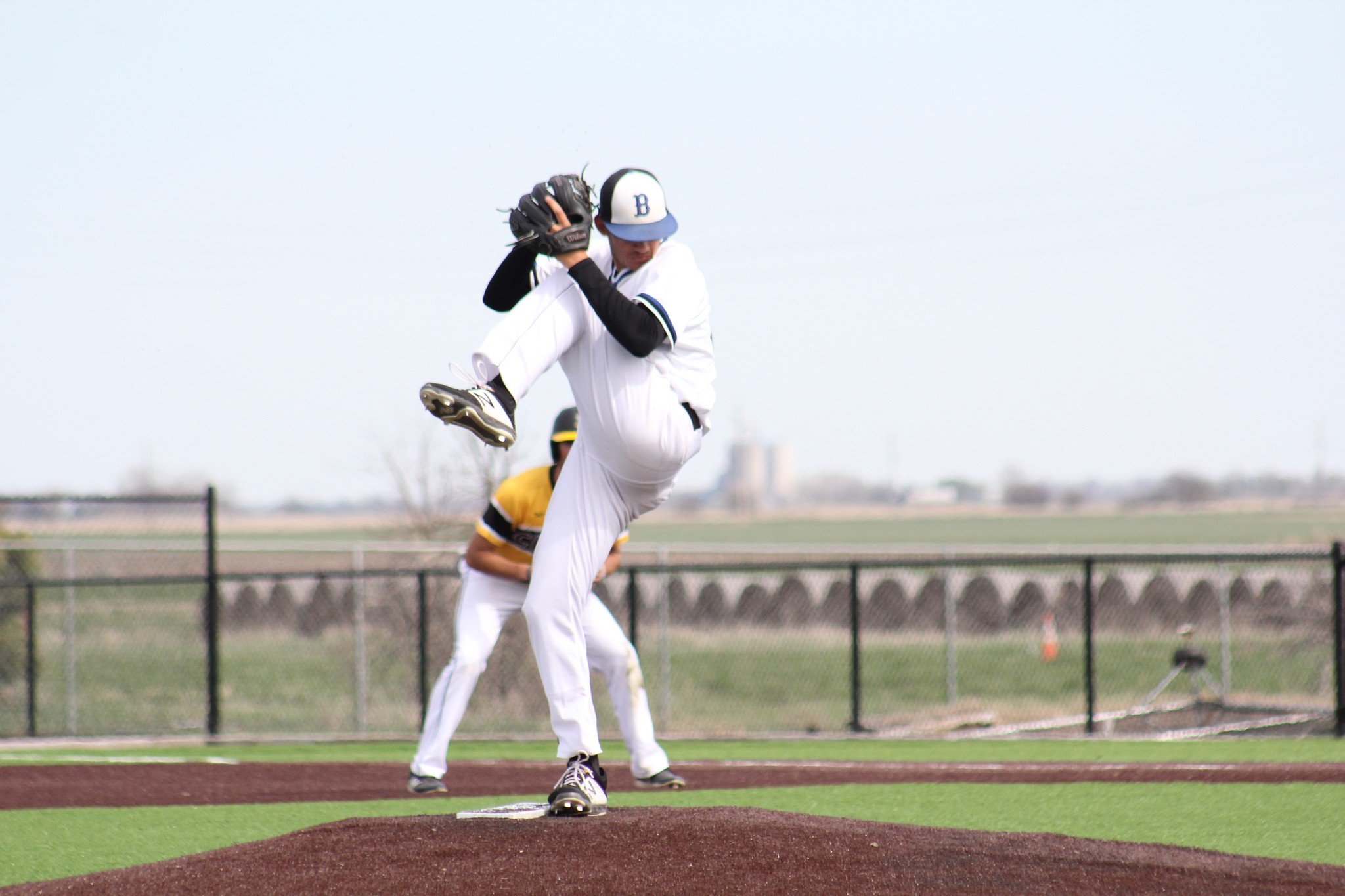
[493,161,601,258]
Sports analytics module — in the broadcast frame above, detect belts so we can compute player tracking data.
[680,400,701,430]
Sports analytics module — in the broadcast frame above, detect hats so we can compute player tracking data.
[549,404,582,444]
[597,168,678,239]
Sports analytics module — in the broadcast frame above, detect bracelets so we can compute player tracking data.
[526,565,532,583]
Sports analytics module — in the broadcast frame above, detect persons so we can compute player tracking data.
[407,407,686,795]
[420,170,714,817]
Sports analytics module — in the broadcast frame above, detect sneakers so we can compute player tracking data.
[418,359,516,449]
[547,751,609,817]
[633,768,687,789]
[406,771,448,794]
